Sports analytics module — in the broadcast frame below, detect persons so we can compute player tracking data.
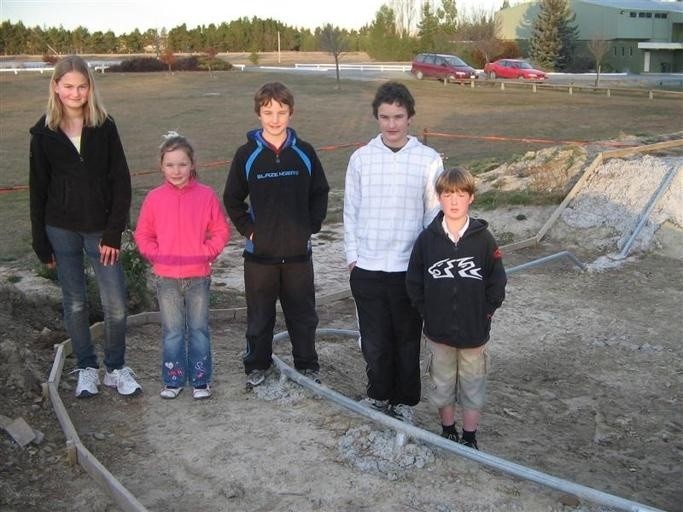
[224,83,329,391]
[29,56,144,396]
[344,82,446,427]
[136,135,230,399]
[407,164,507,450]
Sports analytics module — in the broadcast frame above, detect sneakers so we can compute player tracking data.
[194,384,213,400]
[440,431,458,441]
[161,385,185,399]
[461,439,479,449]
[359,396,393,413]
[246,363,275,387]
[75,367,100,398]
[299,369,322,382]
[393,403,416,423]
[105,368,143,396]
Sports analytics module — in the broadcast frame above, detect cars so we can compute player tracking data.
[484,59,548,83]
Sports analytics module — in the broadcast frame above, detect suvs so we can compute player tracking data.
[411,54,479,84]
